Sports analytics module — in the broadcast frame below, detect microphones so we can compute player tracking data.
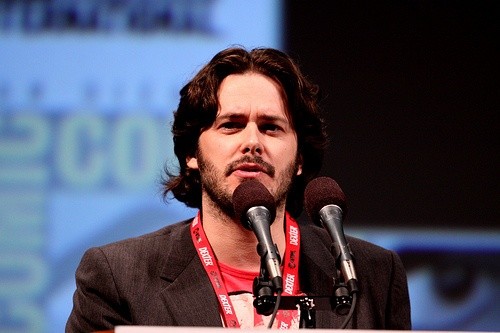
[232,181,283,293]
[305,178,358,288]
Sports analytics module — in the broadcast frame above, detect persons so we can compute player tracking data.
[63,42,412,333]
